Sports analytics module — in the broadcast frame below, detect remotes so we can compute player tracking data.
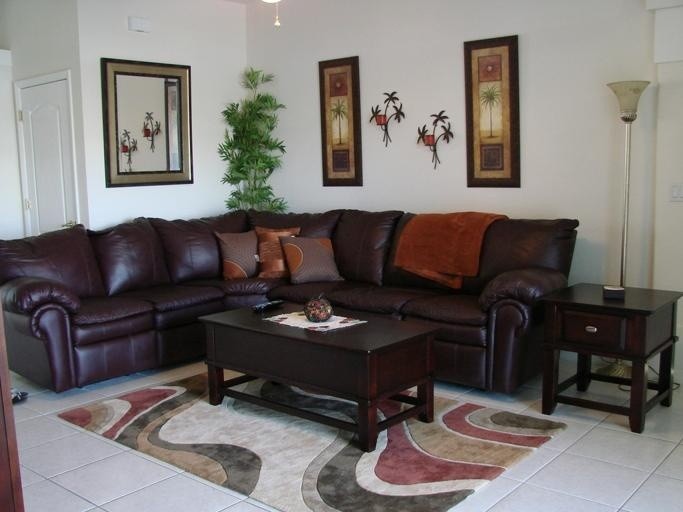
[251,300,284,313]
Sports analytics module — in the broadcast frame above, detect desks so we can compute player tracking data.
[195,296,445,454]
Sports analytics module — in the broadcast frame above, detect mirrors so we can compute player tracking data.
[97,56,194,189]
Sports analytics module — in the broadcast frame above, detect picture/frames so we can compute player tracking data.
[317,54,364,188]
[462,34,522,189]
[163,76,181,173]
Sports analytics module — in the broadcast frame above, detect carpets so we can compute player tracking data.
[52,366,571,512]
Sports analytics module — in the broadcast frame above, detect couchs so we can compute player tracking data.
[1,207,580,395]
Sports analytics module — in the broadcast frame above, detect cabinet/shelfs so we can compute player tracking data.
[536,281,683,434]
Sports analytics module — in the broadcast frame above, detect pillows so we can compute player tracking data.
[280,236,345,286]
[255,227,301,280]
[216,229,260,280]
[211,224,345,287]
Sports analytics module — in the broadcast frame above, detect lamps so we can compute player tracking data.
[140,111,162,153]
[368,90,405,149]
[594,79,655,385]
[416,110,454,171]
[117,129,138,173]
